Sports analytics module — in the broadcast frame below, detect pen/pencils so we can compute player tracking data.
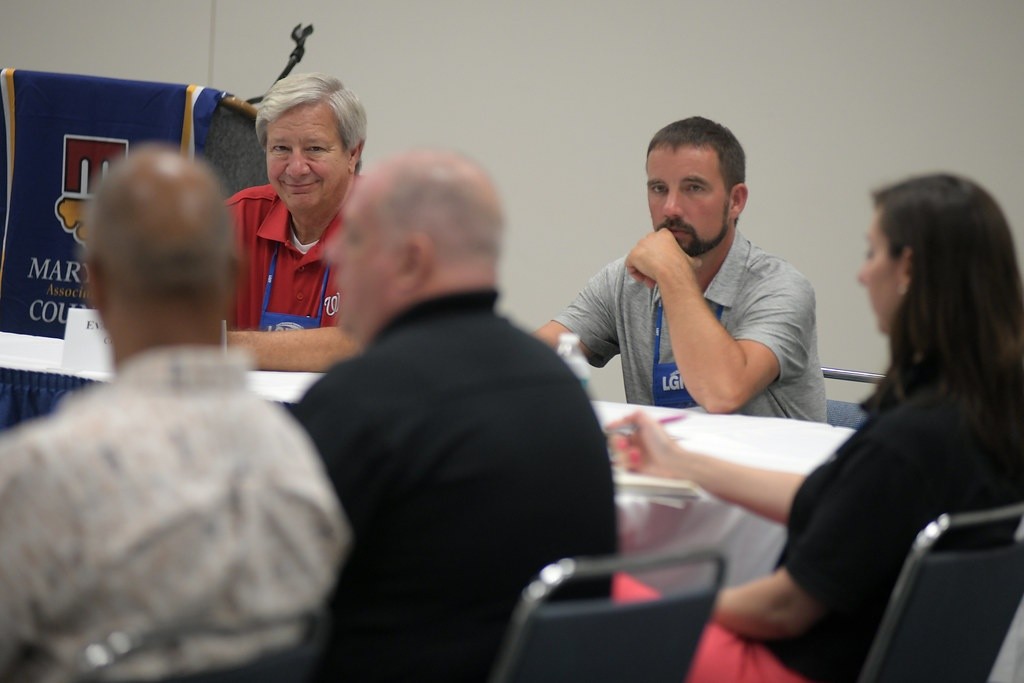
[604,412,688,437]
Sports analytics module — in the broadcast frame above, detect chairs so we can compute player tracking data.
[858,501,1024,683]
[492,545,733,683]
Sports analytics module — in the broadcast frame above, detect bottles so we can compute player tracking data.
[555,334,588,390]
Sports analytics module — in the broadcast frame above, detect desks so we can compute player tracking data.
[0,331,853,518]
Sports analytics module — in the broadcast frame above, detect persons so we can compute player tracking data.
[525,116,827,429]
[282,149,621,683]
[217,71,367,373]
[602,175,1024,683]
[0,143,352,683]
